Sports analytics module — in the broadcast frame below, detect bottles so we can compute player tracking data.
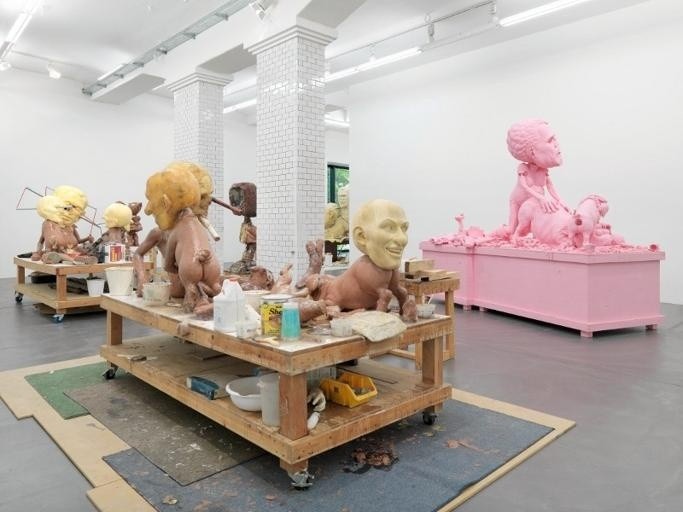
[281,301,302,342]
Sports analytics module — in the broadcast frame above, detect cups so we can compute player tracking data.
[225,363,237,414]
[258,373,282,428]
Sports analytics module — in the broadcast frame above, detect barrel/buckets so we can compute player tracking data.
[257,373,280,426]
[104,267,133,294]
[86,278,104,297]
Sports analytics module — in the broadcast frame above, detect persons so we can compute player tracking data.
[27,160,226,313]
[306,198,420,321]
[498,116,574,241]
[323,204,347,245]
[335,181,349,220]
